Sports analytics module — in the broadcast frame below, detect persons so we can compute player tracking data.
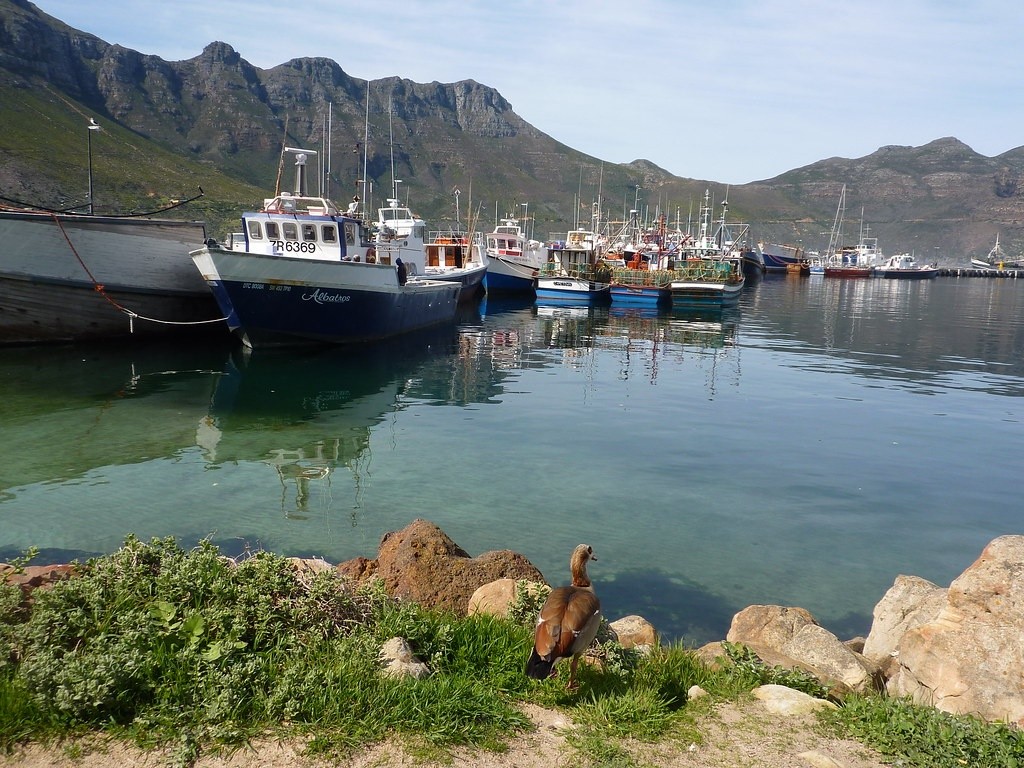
[396,258,407,286]
[705,250,717,256]
[740,246,748,257]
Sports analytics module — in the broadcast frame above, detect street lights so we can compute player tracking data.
[86,118,103,215]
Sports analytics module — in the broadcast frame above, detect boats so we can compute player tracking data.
[422,174,1024,323]
[189,81,462,350]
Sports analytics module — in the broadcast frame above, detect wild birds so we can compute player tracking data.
[524,544,602,690]
